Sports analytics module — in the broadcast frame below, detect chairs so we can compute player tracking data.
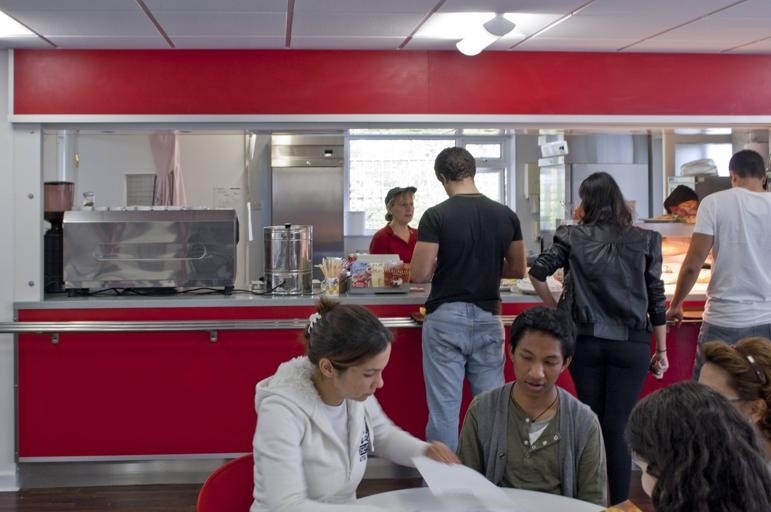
[197,455,253,512]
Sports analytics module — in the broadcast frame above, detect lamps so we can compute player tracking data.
[456,13,516,56]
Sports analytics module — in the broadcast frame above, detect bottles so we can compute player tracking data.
[82,191,93,206]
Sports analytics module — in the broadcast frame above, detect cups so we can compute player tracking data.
[320,278,340,297]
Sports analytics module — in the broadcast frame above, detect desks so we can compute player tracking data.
[358,485,607,512]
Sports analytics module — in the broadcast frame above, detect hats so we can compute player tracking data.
[664,185,698,213]
[385,187,417,204]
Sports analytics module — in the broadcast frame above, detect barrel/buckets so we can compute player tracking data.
[264,223,314,296]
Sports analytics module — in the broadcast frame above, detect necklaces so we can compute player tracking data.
[531,392,559,423]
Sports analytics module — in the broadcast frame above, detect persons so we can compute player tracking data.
[626,336,770,512]
[412,147,528,457]
[249,294,462,511]
[369,186,417,283]
[666,150,769,381]
[454,305,608,507]
[528,173,669,505]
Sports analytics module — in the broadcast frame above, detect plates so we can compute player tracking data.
[638,218,672,222]
[517,282,563,294]
